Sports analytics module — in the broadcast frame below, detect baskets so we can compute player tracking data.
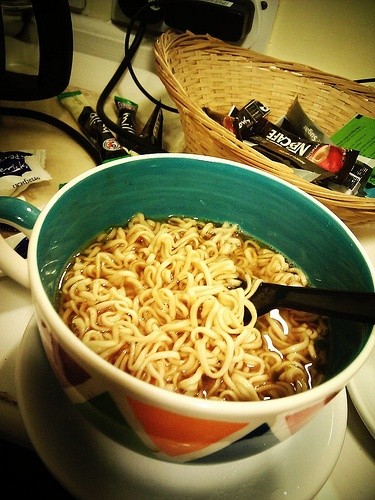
[154,29,374,228]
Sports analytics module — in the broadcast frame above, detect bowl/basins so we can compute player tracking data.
[26,153,375,466]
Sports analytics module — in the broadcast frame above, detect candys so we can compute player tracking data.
[0,148,56,276]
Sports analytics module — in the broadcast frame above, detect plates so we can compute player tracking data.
[14,311,347,500]
[347,237,375,442]
